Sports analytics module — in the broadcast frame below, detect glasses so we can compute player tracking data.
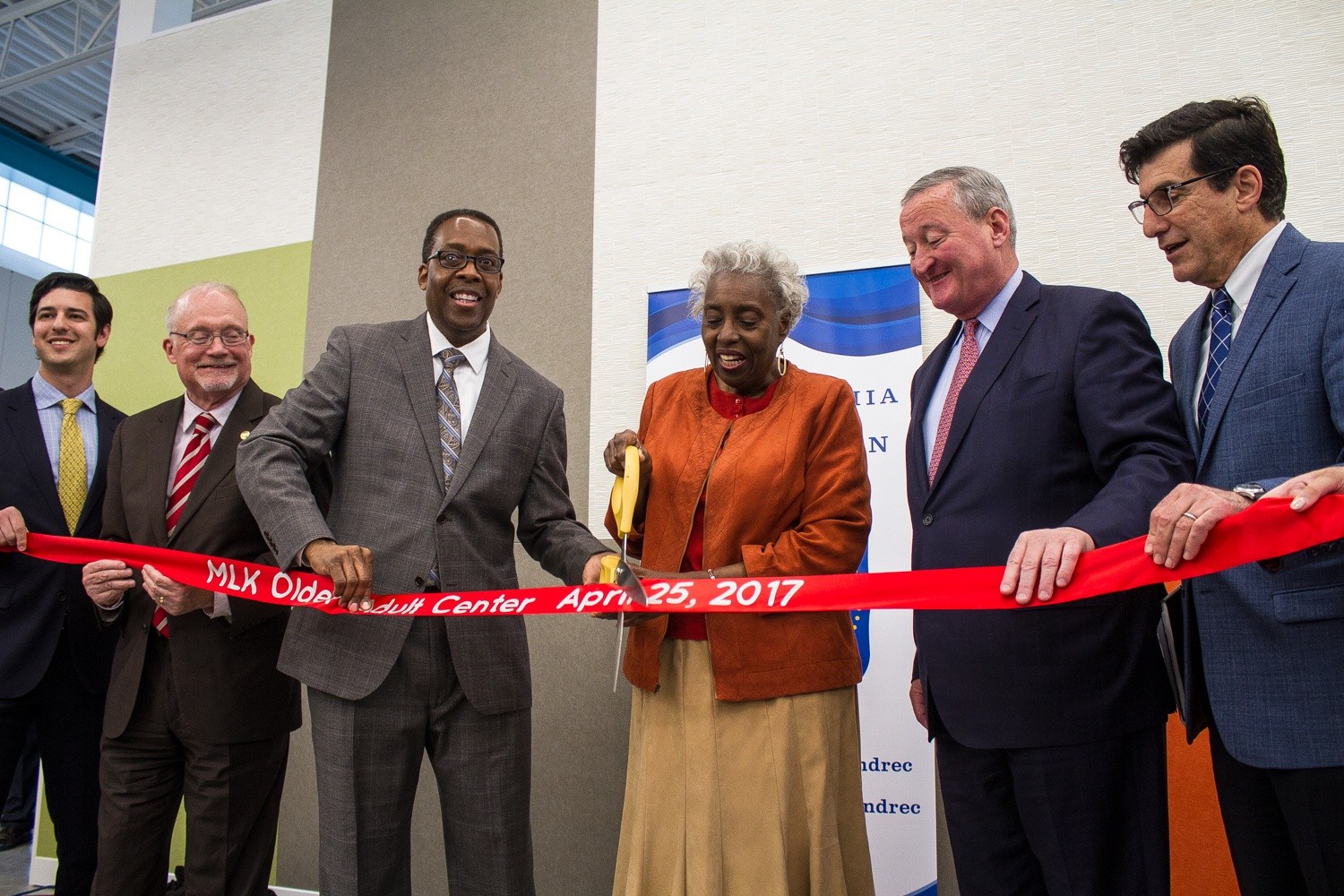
[1129,165,1238,225]
[169,332,250,349]
[426,249,506,274]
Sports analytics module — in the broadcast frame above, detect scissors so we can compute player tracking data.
[599,446,648,694]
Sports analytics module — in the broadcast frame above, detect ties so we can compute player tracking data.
[929,319,979,490]
[1198,286,1235,455]
[152,413,216,639]
[59,398,88,536]
[431,348,461,584]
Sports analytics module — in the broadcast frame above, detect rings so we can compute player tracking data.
[1182,512,1198,521]
[159,596,165,604]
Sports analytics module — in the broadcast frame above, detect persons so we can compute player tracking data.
[898,167,1192,896]
[1256,466,1344,512]
[82,284,334,896]
[236,209,625,896]
[1118,96,1344,896]
[0,272,129,896]
[597,238,876,896]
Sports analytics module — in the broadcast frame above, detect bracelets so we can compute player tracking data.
[706,568,716,580]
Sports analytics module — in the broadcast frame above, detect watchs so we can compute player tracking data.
[1232,483,1265,500]
